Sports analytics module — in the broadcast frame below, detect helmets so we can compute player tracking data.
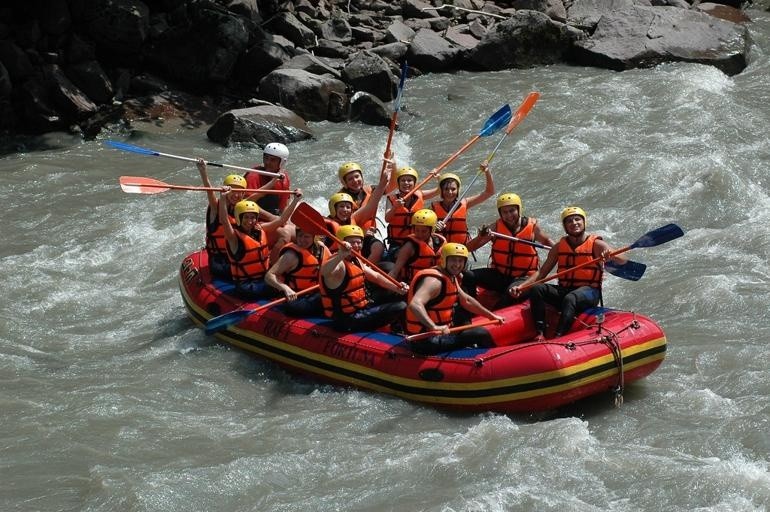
[336,225,364,249]
[412,209,437,232]
[224,174,247,189]
[234,201,259,226]
[396,167,418,188]
[339,162,363,189]
[440,243,468,269]
[328,193,353,218]
[438,173,461,198]
[497,193,522,218]
[263,142,289,169]
[561,207,587,234]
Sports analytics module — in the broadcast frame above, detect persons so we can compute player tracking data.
[319,225,410,333]
[512,205,628,343]
[196,142,304,298]
[463,193,552,319]
[407,242,505,351]
[326,149,441,263]
[430,160,496,244]
[264,226,332,317]
[389,209,448,283]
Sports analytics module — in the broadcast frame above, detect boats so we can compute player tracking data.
[177,246,667,417]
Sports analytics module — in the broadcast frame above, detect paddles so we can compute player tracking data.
[289,201,405,289]
[119,176,301,196]
[380,61,406,180]
[511,225,684,289]
[401,105,512,204]
[488,229,646,282]
[432,93,540,232]
[105,140,285,179]
[393,318,503,351]
[207,283,321,333]
